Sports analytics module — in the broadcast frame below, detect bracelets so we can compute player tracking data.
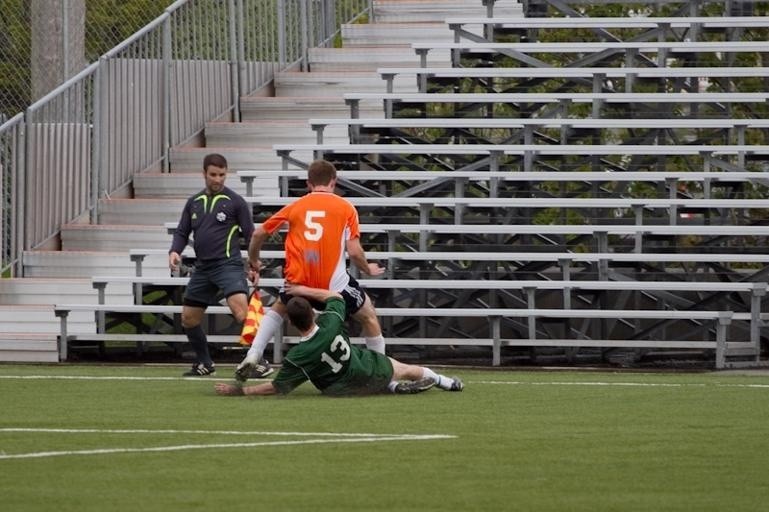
[228,383,247,396]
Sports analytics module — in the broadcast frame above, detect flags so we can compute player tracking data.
[239,287,267,348]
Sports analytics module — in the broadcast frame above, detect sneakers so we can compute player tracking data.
[235,359,274,382]
[182,362,216,376]
[395,378,435,394]
[450,376,464,391]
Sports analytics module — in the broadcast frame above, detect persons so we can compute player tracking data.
[212,282,465,397]
[167,152,274,379]
[232,158,387,386]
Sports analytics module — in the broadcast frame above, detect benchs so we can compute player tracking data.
[55,172,768,372]
[407,0,768,66]
[234,68,768,174]
